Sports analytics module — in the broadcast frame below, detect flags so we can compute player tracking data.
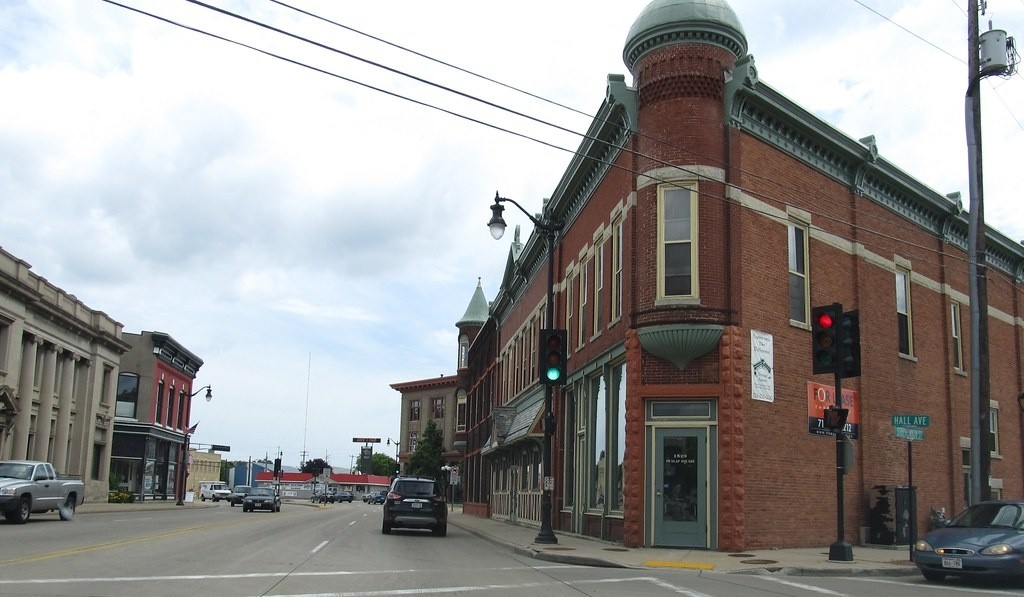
[186,424,198,434]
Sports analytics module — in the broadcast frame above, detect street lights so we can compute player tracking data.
[386,438,400,484]
[175,385,212,504]
[486,190,561,544]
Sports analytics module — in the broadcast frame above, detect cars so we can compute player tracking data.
[243,486,282,513]
[332,491,353,503]
[231,485,253,507]
[363,490,386,505]
[912,499,1024,582]
[310,490,335,504]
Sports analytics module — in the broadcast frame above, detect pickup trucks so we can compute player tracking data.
[0,460,85,525]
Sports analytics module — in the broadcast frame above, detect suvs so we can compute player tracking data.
[382,477,449,537]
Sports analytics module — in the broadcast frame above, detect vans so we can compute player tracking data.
[199,483,231,502]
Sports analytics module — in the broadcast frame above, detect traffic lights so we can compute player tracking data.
[811,301,839,376]
[823,407,849,432]
[538,328,569,387]
[395,464,400,474]
